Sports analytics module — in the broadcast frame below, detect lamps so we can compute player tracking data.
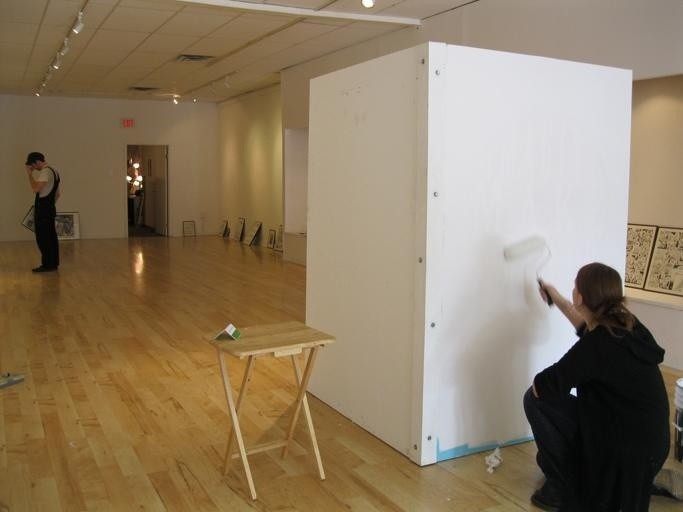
[34,1,90,98]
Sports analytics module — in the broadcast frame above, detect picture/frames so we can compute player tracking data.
[21,205,37,234]
[54,211,81,240]
[624,223,659,289]
[183,221,196,237]
[218,217,284,252]
[643,224,683,297]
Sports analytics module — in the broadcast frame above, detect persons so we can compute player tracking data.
[23,151,62,273]
[522,262,672,511]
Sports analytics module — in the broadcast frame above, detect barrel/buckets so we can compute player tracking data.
[670,378,683,462]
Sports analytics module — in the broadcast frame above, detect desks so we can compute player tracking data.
[200,320,337,501]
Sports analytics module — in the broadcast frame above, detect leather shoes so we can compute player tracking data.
[31,263,59,273]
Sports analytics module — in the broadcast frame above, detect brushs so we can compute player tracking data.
[503,236,554,309]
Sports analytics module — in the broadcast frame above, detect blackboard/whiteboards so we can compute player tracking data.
[284,127,309,238]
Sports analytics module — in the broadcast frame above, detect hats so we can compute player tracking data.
[24,152,45,166]
